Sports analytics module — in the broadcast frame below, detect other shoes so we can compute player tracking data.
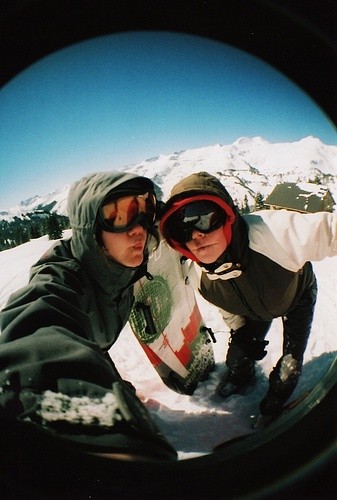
[216,362,256,398]
[260,366,300,413]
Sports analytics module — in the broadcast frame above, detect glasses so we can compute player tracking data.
[97,188,158,233]
[164,199,227,243]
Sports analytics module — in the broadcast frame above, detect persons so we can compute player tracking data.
[1,171,169,470]
[161,171,337,425]
